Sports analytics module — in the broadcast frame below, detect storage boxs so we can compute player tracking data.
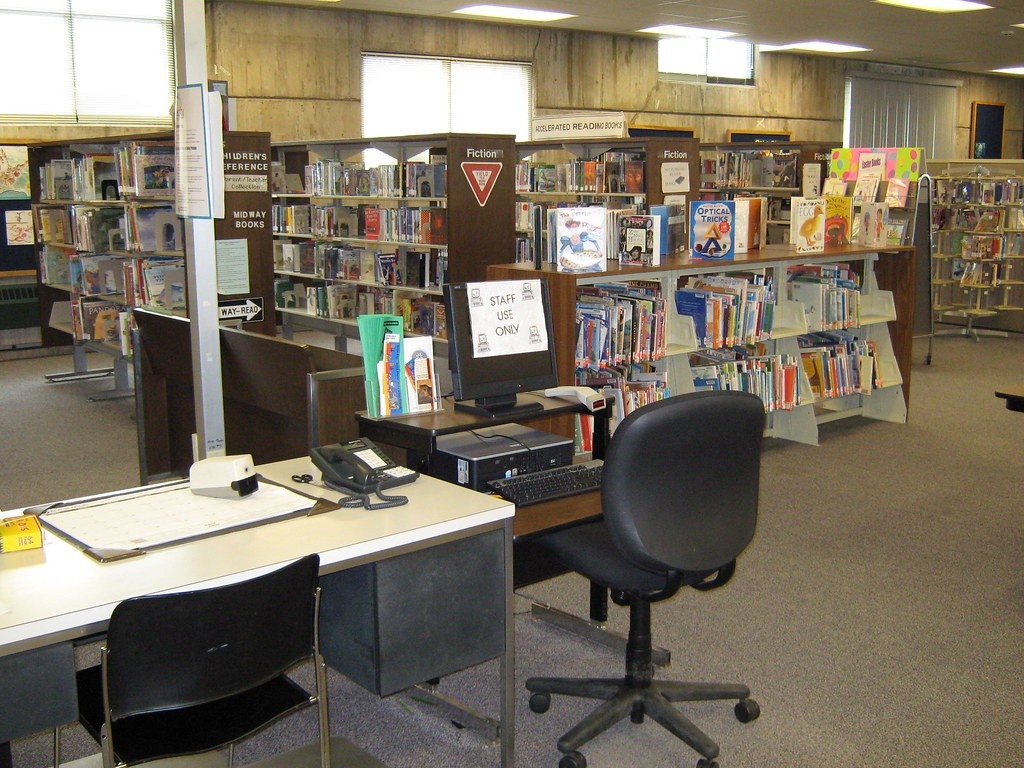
[0,514,43,554]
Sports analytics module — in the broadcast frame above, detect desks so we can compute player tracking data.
[354,395,616,622]
[0,456,515,768]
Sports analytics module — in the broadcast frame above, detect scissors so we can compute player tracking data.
[292,474,333,491]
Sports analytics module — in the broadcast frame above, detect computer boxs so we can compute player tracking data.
[431,423,573,493]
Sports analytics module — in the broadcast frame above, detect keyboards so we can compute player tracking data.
[487,459,604,508]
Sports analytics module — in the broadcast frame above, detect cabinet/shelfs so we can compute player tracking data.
[27,132,1024,444]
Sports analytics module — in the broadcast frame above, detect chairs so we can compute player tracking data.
[54,553,331,768]
[525,390,765,768]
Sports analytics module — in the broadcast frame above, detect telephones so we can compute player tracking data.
[310,437,420,494]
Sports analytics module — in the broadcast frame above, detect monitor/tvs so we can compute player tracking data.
[442,278,560,417]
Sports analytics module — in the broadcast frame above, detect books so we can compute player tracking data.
[31,140,187,357]
[515,151,919,272]
[930,178,1024,285]
[271,158,447,340]
[575,262,881,457]
